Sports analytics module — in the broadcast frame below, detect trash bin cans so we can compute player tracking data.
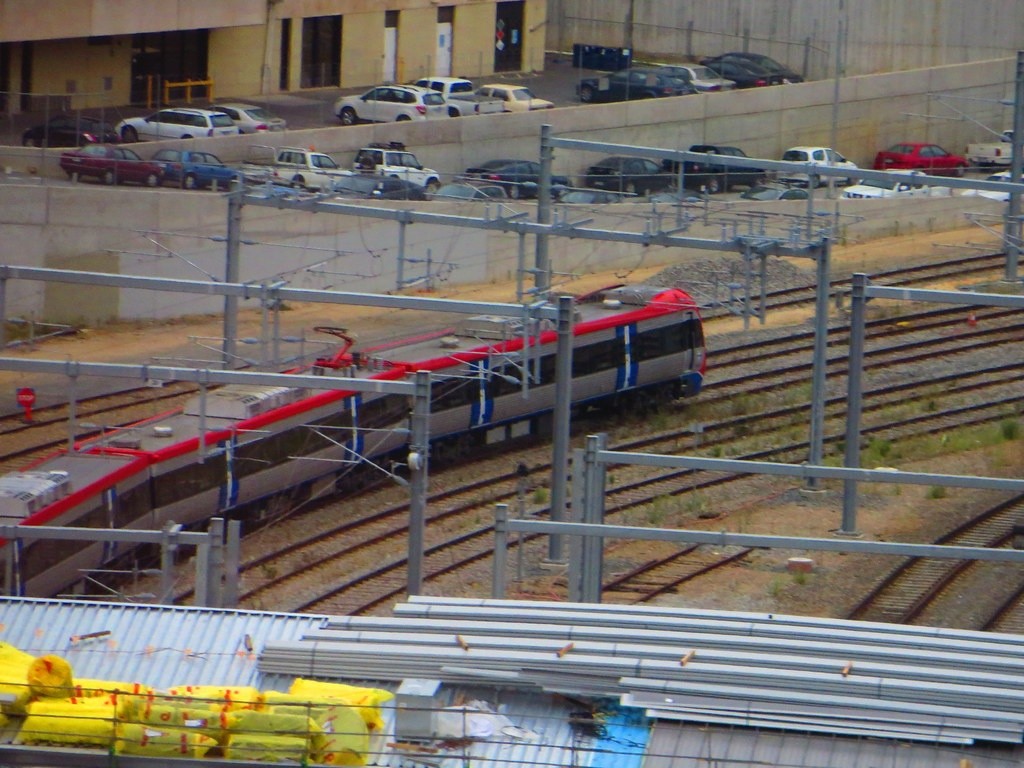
[572,44,632,71]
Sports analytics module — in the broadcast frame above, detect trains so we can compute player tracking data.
[0,284,707,601]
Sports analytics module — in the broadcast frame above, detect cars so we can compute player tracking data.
[465,158,574,199]
[777,146,865,188]
[650,187,709,201]
[204,102,288,136]
[655,62,737,92]
[735,184,816,200]
[474,84,554,113]
[962,171,1024,201]
[334,173,432,201]
[22,116,123,148]
[585,156,683,196]
[150,148,247,190]
[874,143,970,177]
[838,167,954,201]
[698,52,804,89]
[59,145,168,187]
[430,183,510,201]
[553,188,625,205]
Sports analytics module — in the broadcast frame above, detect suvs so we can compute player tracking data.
[334,83,450,125]
[352,141,441,194]
[575,66,693,102]
[114,108,240,143]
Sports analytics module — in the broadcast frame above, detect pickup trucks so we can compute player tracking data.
[661,144,768,194]
[410,76,506,117]
[964,129,1015,166]
[235,144,355,192]
